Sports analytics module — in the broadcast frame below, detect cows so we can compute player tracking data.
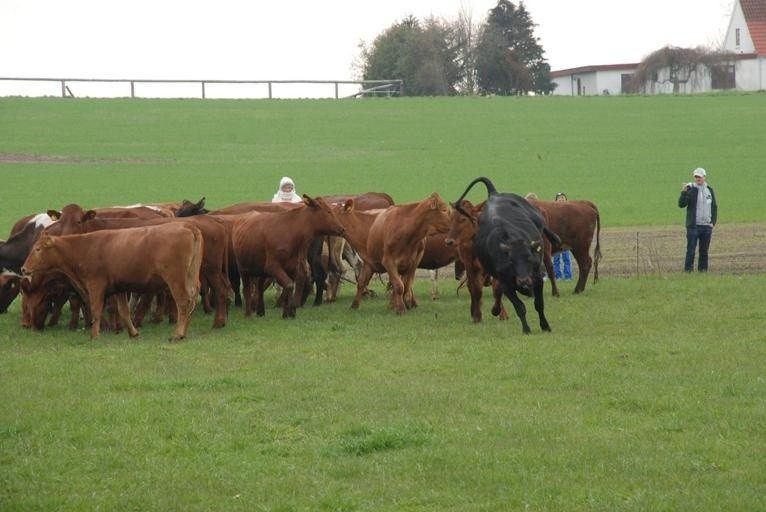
[1,189,445,343]
[445,175,603,335]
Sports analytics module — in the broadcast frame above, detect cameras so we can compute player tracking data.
[686,182,693,190]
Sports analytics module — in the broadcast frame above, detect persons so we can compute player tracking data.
[525,192,537,199]
[678,168,718,273]
[272,177,302,203]
[553,192,573,282]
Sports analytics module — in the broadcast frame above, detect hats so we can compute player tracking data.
[693,167,706,178]
